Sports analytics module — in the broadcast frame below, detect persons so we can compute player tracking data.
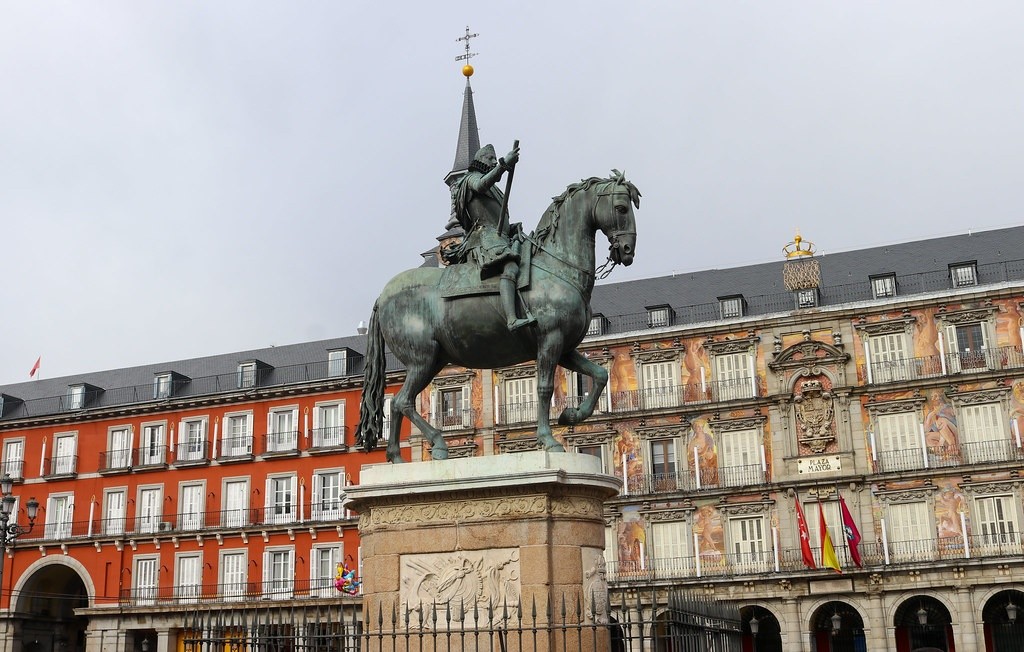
[458,144,535,332]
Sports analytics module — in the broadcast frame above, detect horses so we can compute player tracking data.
[353,169,642,464]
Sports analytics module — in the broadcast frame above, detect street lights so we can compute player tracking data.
[0,471,39,597]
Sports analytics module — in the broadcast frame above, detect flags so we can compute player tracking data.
[819,501,842,575]
[794,493,817,571]
[29,356,40,377]
[840,493,863,569]
